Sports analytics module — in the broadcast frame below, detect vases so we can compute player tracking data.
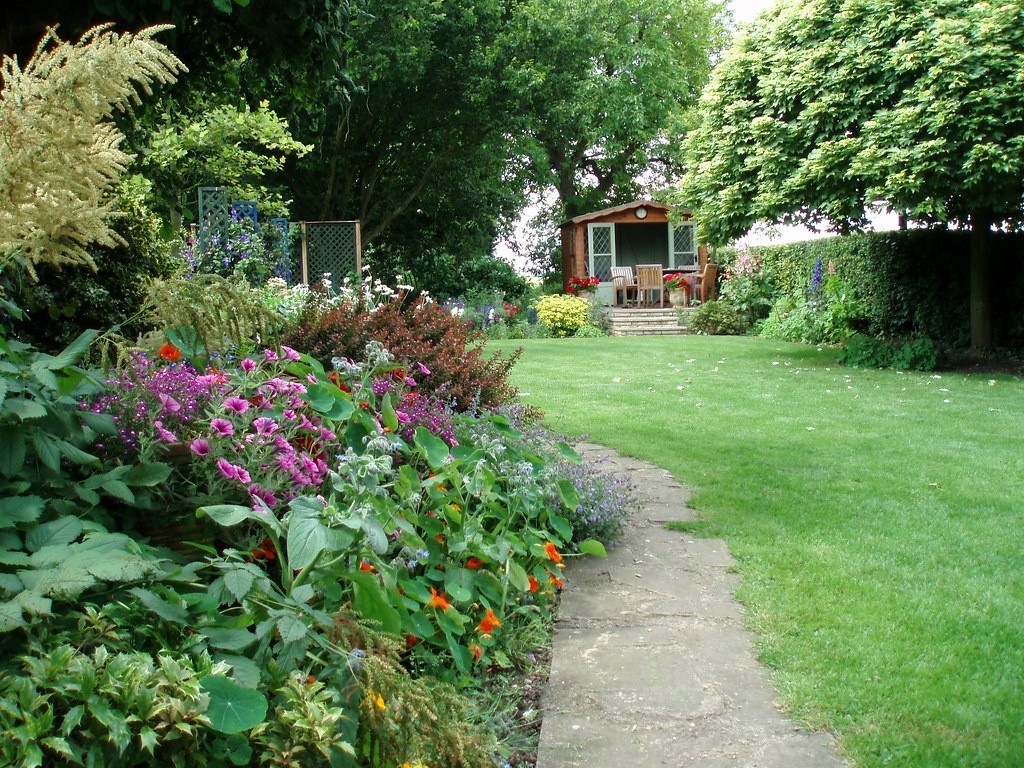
[669,288,685,307]
[577,291,595,298]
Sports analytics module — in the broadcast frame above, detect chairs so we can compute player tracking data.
[637,264,663,309]
[691,263,719,303]
[610,266,637,308]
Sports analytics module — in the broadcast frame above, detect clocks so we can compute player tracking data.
[635,208,647,219]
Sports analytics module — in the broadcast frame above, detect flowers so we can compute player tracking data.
[564,273,599,293]
[666,271,692,293]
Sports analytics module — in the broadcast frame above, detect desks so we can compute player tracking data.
[649,268,701,308]
